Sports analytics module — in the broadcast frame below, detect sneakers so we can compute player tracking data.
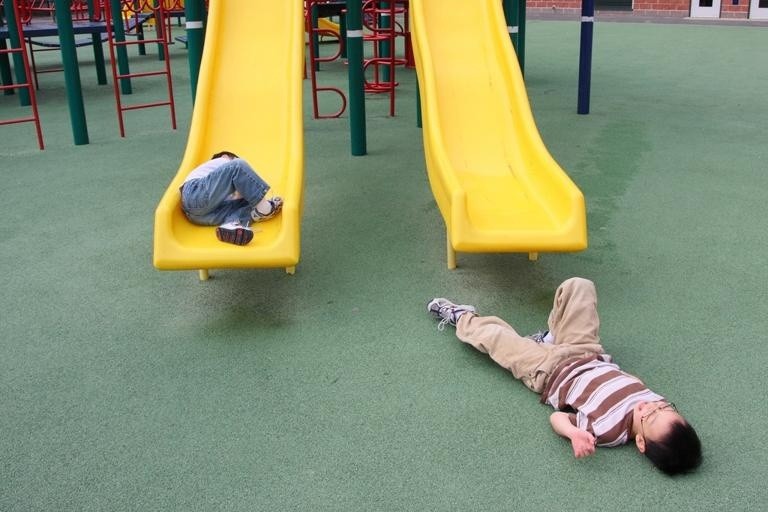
[248,196,283,223]
[215,221,254,246]
[425,298,476,331]
[522,329,550,343]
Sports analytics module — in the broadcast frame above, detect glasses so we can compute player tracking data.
[641,402,678,440]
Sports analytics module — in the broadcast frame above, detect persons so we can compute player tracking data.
[178,151,283,246]
[426,277,703,477]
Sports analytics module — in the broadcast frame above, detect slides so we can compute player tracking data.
[121,0,341,37]
[151,0,305,270]
[408,1,588,253]
